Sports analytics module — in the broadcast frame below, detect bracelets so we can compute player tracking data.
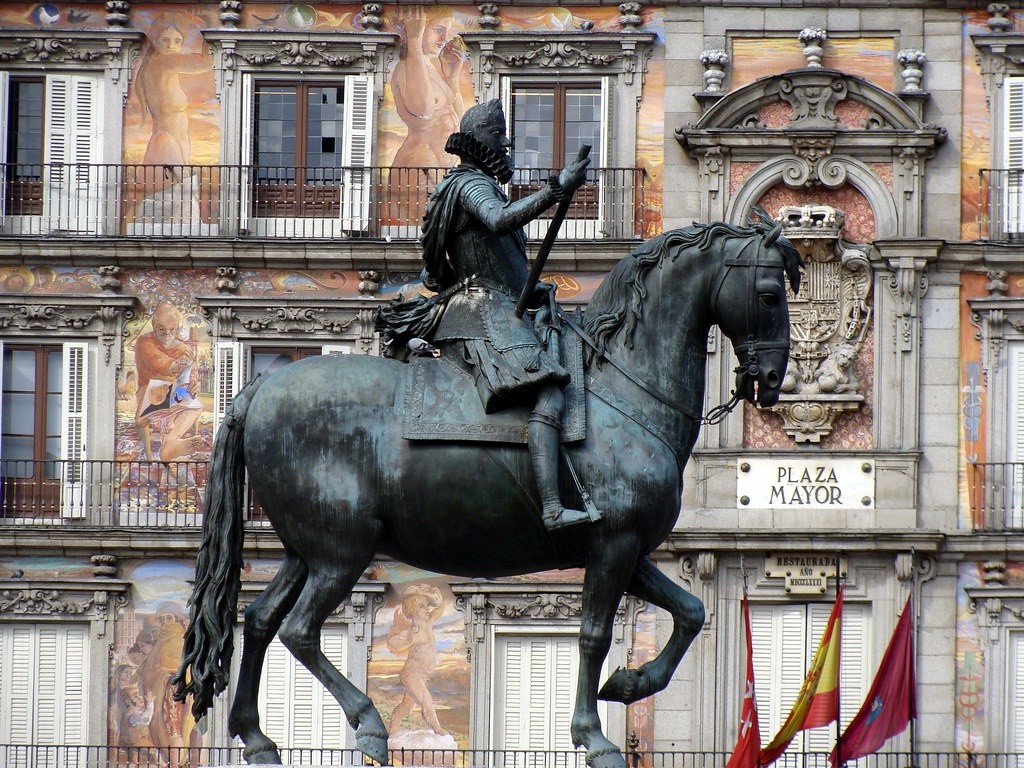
[548,175,566,202]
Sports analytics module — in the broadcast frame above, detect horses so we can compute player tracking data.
[170,205,805,768]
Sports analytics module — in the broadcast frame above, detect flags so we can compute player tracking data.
[827,593,917,768]
[760,580,844,768]
[724,593,761,768]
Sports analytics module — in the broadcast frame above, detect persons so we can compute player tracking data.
[418,99,605,532]
